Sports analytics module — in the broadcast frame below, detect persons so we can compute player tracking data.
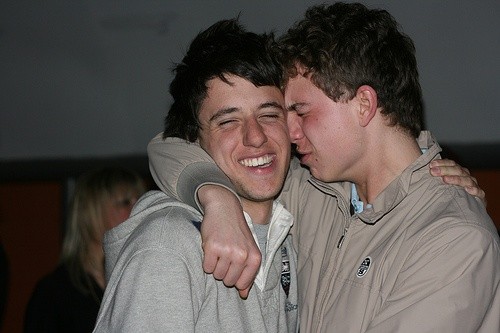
[21,162,147,333]
[91,18,485,333]
[146,1,500,333]
